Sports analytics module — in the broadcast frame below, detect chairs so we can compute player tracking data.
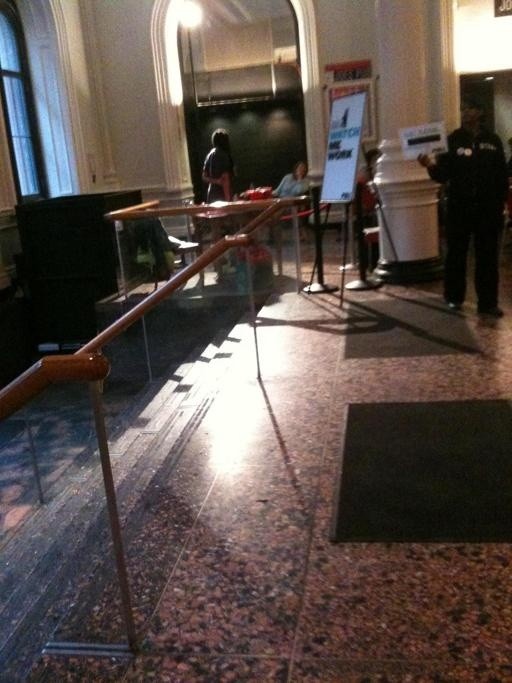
[142,208,200,290]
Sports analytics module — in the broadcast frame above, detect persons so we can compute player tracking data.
[270,159,312,197]
[416,96,510,318]
[199,126,241,269]
[134,212,199,283]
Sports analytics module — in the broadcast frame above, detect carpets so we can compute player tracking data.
[331,398,511,544]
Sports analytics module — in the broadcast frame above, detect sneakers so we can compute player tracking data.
[445,295,466,311]
[357,274,388,293]
[474,298,507,320]
